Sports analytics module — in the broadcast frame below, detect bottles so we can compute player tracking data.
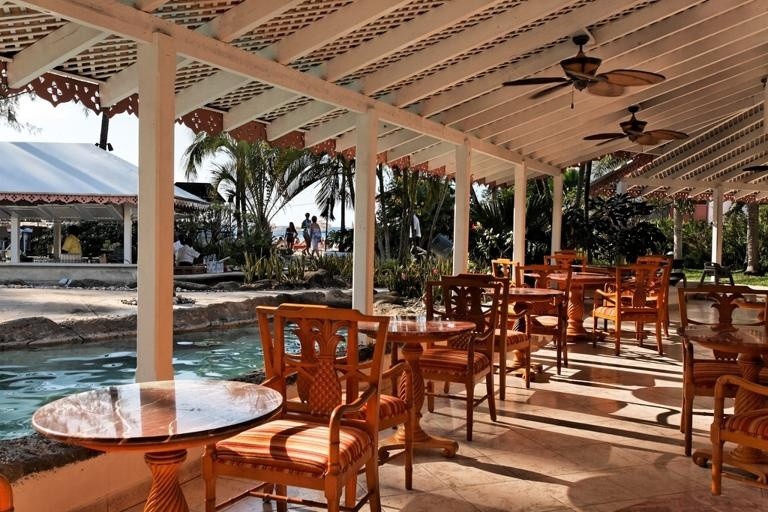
[60,253,82,263]
[202,253,217,268]
[88,253,93,263]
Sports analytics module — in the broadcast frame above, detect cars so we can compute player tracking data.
[294,237,326,253]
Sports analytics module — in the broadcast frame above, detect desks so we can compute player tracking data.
[30,379,283,512]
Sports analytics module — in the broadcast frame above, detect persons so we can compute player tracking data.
[406,207,422,246]
[176,238,201,266]
[174,233,186,261]
[309,216,322,257]
[301,212,312,256]
[61,225,83,257]
[284,221,298,250]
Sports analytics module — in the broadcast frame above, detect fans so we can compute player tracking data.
[501,32,665,100]
[583,107,688,146]
